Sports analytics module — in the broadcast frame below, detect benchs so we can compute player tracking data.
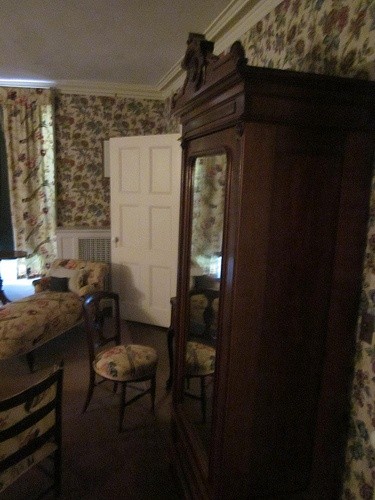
[0,259,110,373]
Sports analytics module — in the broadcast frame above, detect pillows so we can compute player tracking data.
[49,266,86,294]
[49,276,70,292]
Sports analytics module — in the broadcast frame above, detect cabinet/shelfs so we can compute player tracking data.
[166,32,375,500]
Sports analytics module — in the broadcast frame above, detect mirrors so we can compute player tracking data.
[177,145,233,479]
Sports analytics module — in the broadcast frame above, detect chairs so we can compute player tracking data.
[82,291,158,432]
[0,360,64,500]
[185,294,216,423]
